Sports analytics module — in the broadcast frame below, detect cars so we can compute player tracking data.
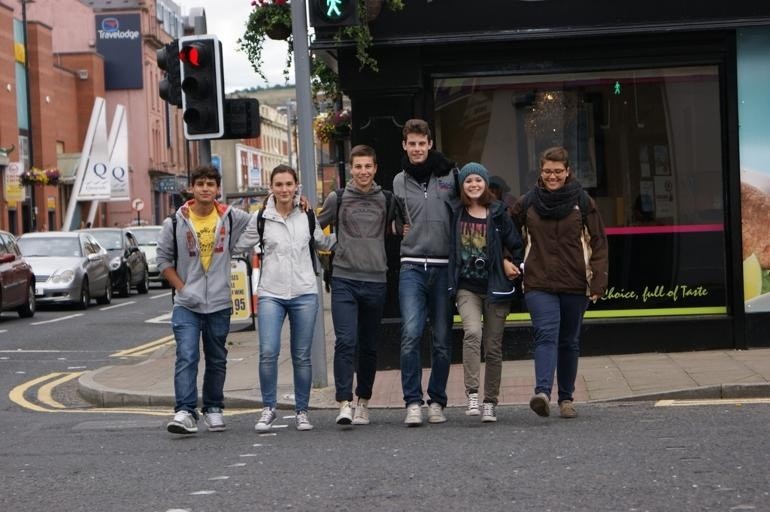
[0,230,36,317]
[72,228,151,295]
[16,232,111,308]
[125,225,169,288]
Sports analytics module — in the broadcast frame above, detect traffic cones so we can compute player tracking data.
[250,255,262,316]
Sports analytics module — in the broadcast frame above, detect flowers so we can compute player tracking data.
[314,109,352,143]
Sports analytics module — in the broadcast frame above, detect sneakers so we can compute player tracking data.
[404,402,422,424]
[530,394,550,416]
[482,403,497,422]
[167,410,198,435]
[354,404,370,424]
[426,402,446,423]
[337,403,352,425]
[466,392,481,416]
[295,411,313,431]
[560,400,576,417]
[202,411,226,432]
[254,406,277,433]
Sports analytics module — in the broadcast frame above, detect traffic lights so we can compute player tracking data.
[310,1,356,28]
[223,101,254,136]
[154,41,179,103]
[179,38,223,141]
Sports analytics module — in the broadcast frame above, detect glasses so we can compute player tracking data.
[541,167,565,177]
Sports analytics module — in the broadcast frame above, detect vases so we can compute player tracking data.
[331,125,350,139]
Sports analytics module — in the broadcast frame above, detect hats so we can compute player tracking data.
[458,162,490,186]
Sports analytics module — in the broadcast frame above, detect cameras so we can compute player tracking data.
[468,256,489,272]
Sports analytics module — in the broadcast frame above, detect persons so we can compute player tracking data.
[231,164,338,432]
[402,163,524,423]
[316,145,396,425]
[391,119,461,427]
[488,171,521,207]
[156,165,311,433]
[503,146,609,417]
